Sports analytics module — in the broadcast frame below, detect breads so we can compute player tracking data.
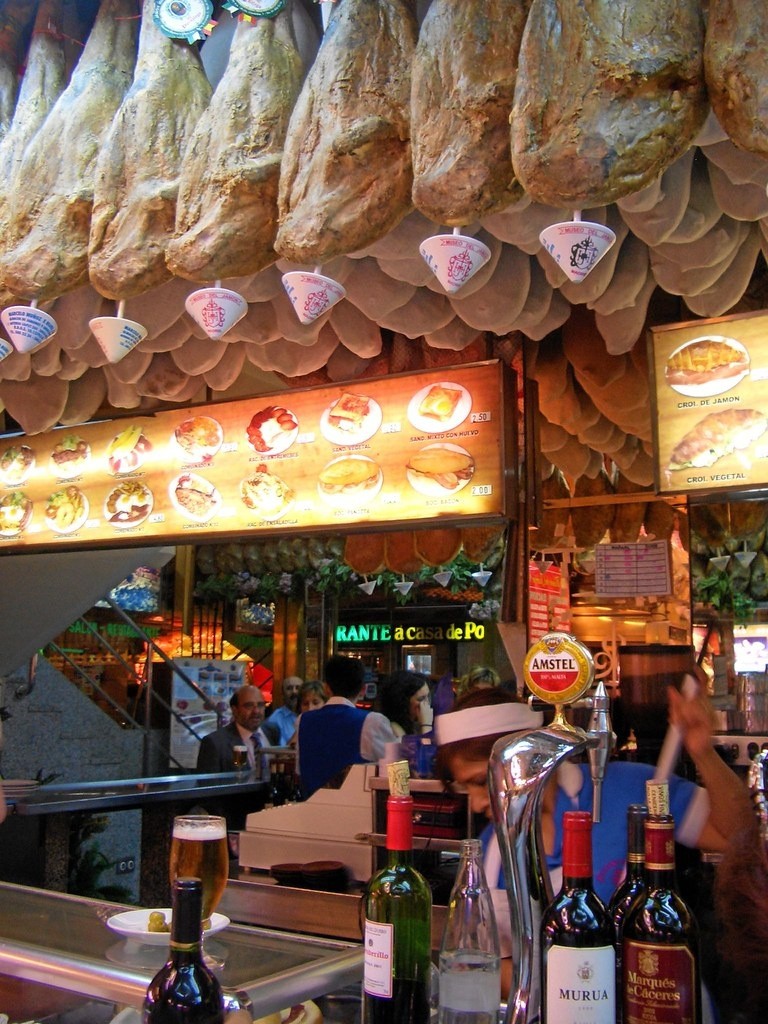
[669,409,768,471]
[318,458,379,485]
[666,339,746,372]
[408,447,471,474]
[418,385,462,421]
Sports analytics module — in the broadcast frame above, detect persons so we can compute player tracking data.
[457,668,501,698]
[259,676,304,747]
[435,664,758,1024]
[355,670,434,779]
[295,654,399,799]
[195,685,278,835]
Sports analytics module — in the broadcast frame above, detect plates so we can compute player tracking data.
[106,908,229,944]
[172,417,223,462]
[105,432,147,475]
[239,473,294,521]
[320,395,382,445]
[270,861,348,892]
[408,383,472,433]
[106,939,228,970]
[318,455,383,506]
[407,443,474,495]
[2,780,39,800]
[0,493,33,536]
[0,445,35,485]
[246,407,299,455]
[666,335,749,397]
[46,491,90,533]
[105,482,154,528]
[50,439,91,478]
[168,473,222,523]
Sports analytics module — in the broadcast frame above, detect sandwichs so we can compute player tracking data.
[328,393,370,432]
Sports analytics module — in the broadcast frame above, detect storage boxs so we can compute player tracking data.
[411,796,467,840]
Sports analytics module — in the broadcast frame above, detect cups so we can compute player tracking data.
[170,815,229,968]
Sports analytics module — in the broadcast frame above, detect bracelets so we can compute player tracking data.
[420,724,432,727]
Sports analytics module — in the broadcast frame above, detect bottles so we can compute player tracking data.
[438,839,501,1024]
[540,811,616,1024]
[364,795,432,1024]
[620,815,702,1024]
[141,877,225,1024]
[608,805,647,1024]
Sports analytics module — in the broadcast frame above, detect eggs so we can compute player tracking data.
[432,400,451,415]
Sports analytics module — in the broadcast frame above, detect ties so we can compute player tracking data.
[250,733,268,779]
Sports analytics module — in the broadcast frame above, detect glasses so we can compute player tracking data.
[238,703,265,710]
